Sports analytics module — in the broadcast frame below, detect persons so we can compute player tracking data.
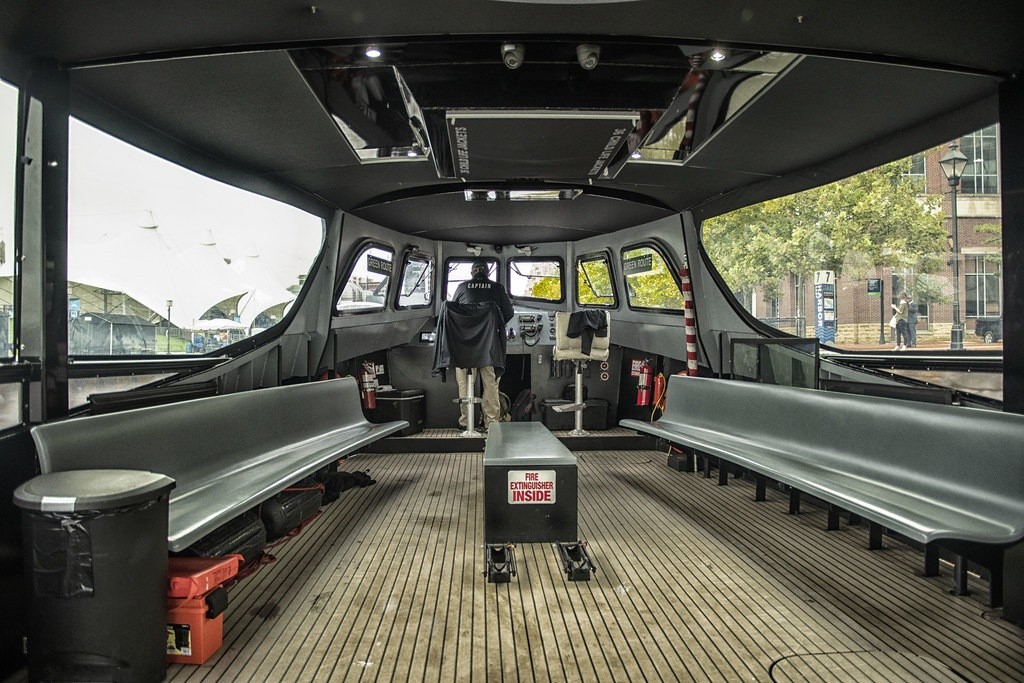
[204,333,208,353]
[452,261,514,433]
[891,292,918,351]
[208,334,218,345]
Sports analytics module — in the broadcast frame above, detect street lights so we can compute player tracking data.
[938,140,969,350]
[164,299,173,354]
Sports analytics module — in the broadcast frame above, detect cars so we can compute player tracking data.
[973,310,1003,343]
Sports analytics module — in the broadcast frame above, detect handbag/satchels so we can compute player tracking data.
[889,314,897,328]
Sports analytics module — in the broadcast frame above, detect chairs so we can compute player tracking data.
[437,301,498,437]
[553,310,612,435]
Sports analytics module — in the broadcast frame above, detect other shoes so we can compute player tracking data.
[457,424,467,432]
[484,428,489,433]
[906,345,911,347]
[892,346,900,351]
[899,345,907,351]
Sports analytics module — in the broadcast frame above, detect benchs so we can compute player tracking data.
[619,375,1024,621]
[26,374,411,554]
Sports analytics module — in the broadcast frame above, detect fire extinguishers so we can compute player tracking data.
[652,373,666,406]
[634,357,654,406]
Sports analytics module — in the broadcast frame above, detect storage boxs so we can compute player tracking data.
[366,388,426,437]
[539,399,609,430]
[167,555,240,664]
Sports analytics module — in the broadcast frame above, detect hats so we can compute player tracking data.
[471,261,488,273]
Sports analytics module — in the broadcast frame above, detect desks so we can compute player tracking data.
[485,421,596,585]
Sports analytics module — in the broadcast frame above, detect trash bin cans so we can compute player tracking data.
[14,468,177,683]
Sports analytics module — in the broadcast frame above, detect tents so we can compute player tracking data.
[185,319,247,352]
